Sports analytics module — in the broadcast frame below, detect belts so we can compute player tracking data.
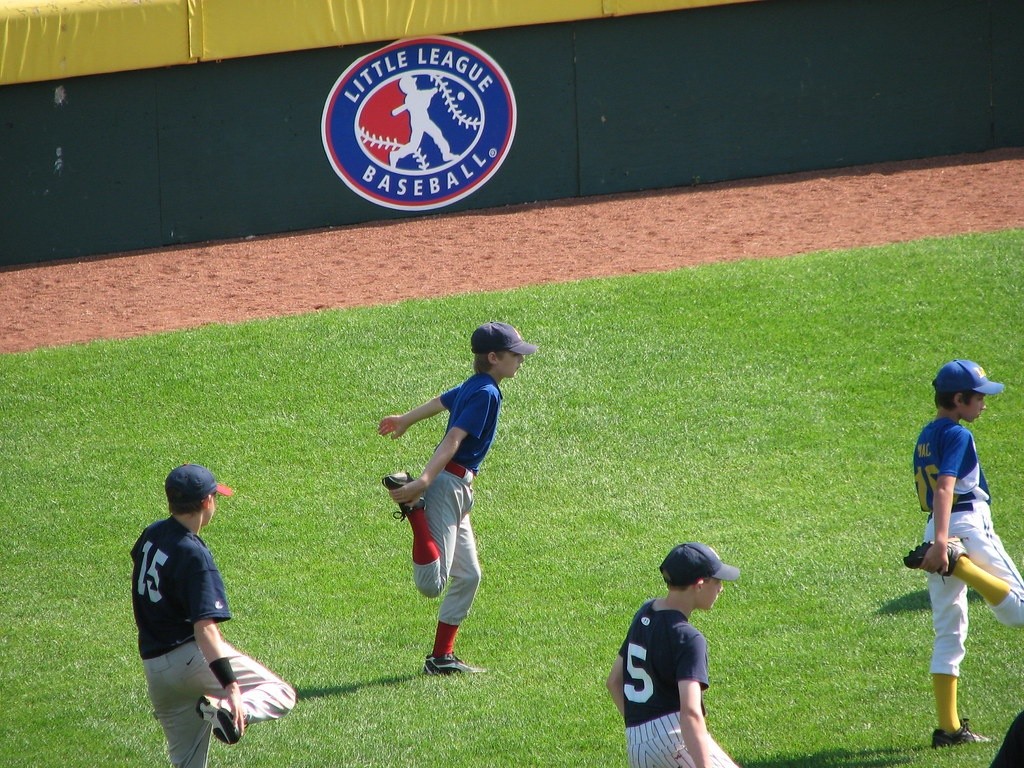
[925,502,974,524]
[443,461,476,484]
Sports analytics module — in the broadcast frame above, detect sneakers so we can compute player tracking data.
[423,652,484,677]
[195,694,240,745]
[931,718,995,747]
[382,470,430,521]
[903,535,970,584]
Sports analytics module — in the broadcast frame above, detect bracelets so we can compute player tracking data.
[208,656,239,690]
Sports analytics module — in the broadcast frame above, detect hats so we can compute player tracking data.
[164,463,233,501]
[471,320,537,355]
[659,543,741,585]
[931,359,1006,394]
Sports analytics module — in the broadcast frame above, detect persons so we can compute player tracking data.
[129,462,297,767]
[903,359,1024,750]
[605,541,740,768]
[377,322,537,677]
